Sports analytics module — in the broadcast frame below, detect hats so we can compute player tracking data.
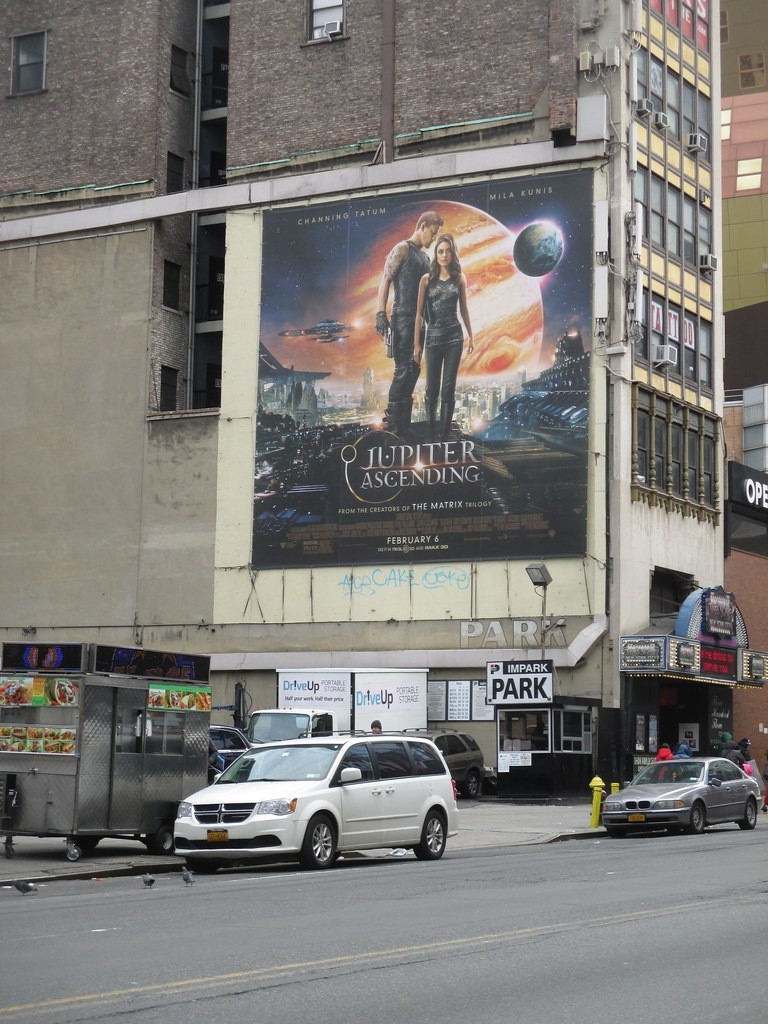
[742,738,752,745]
[371,720,382,729]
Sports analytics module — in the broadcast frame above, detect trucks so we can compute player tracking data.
[243,666,428,759]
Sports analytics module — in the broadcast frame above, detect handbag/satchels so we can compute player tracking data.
[742,761,752,776]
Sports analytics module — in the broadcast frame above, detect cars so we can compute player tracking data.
[601,756,761,838]
[171,728,460,871]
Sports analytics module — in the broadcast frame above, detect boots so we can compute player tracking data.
[382,401,406,446]
[424,396,437,449]
[439,400,455,444]
[402,397,415,438]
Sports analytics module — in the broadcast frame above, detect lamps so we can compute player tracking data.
[526,563,553,660]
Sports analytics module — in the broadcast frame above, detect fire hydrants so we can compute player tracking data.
[588,776,606,828]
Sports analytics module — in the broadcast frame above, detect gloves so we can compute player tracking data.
[376,311,389,336]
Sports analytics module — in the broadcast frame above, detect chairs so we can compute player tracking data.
[664,769,683,780]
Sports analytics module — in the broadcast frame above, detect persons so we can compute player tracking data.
[672,743,693,758]
[720,740,748,768]
[373,210,444,437]
[717,732,733,757]
[529,721,546,750]
[761,749,768,813]
[370,720,383,734]
[655,742,674,760]
[735,737,752,762]
[412,232,476,454]
[311,718,326,736]
[207,736,219,786]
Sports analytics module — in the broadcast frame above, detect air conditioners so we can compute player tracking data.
[636,98,654,116]
[653,112,670,128]
[700,253,718,273]
[688,133,707,152]
[325,21,342,36]
[653,345,678,365]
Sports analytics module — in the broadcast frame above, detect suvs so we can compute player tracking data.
[393,729,488,799]
